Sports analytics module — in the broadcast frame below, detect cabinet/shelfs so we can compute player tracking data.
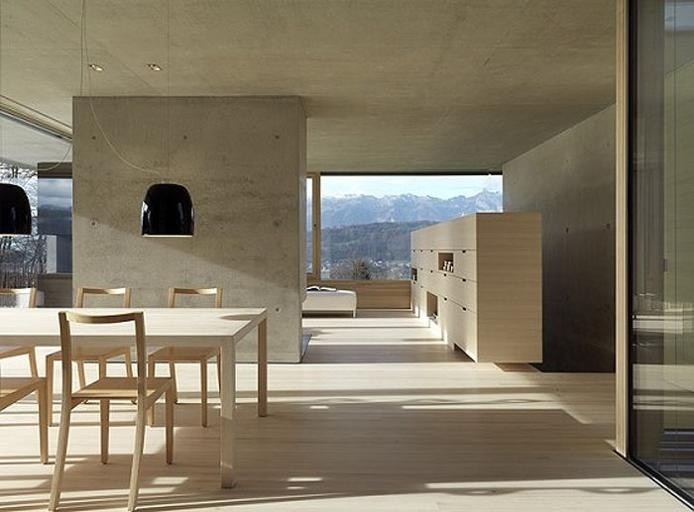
[410,211,541,364]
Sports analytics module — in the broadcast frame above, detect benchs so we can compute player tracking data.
[303,286,357,317]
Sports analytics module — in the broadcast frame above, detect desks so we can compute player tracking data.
[1,309,267,489]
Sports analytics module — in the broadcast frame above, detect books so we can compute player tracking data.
[306,286,336,292]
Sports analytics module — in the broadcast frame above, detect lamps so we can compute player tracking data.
[1,181,32,236]
[135,0,197,241]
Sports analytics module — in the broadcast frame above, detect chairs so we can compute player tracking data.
[1,289,41,403]
[46,285,134,428]
[1,375,48,471]
[46,311,176,510]
[148,287,226,426]
